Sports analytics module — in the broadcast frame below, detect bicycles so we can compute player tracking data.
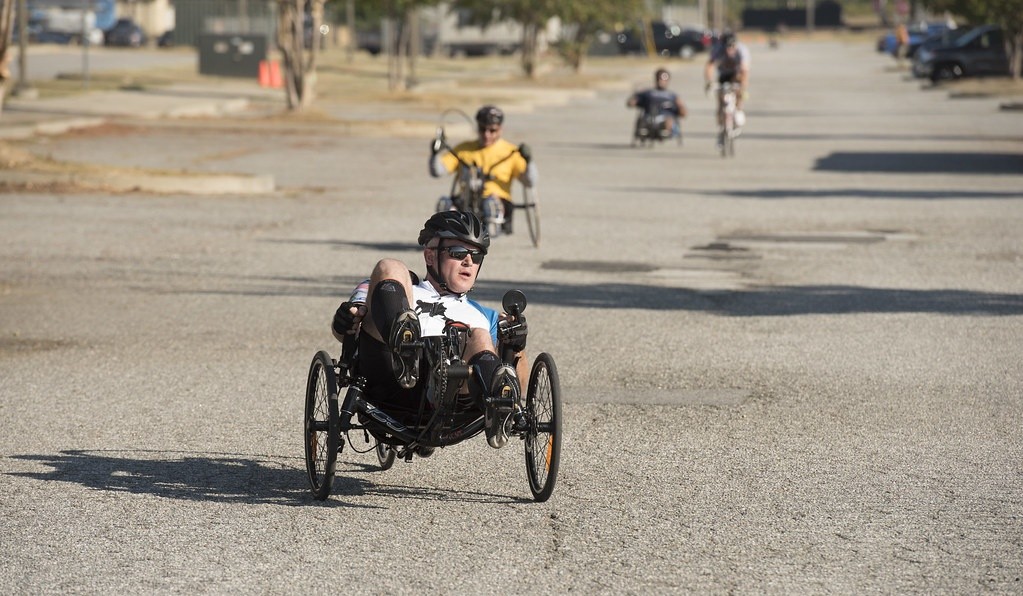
[707,78,744,156]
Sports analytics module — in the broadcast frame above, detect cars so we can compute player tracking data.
[619,22,711,58]
[880,24,952,56]
[915,26,1022,82]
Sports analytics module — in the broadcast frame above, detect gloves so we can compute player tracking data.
[519,143,532,164]
[496,310,528,353]
[429,138,445,156]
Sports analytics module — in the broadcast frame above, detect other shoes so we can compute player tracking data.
[734,111,745,128]
[717,129,725,149]
[484,362,522,449]
[388,308,421,389]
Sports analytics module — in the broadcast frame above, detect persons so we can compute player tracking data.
[332,210,528,449]
[706,32,749,125]
[627,69,686,138]
[429,106,538,237]
[897,22,908,68]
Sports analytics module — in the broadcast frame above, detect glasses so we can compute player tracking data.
[427,245,485,265]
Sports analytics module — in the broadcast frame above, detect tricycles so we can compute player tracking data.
[630,100,682,149]
[303,289,563,500]
[433,131,542,248]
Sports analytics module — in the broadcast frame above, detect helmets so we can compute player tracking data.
[721,33,735,48]
[418,210,490,254]
[475,107,503,125]
